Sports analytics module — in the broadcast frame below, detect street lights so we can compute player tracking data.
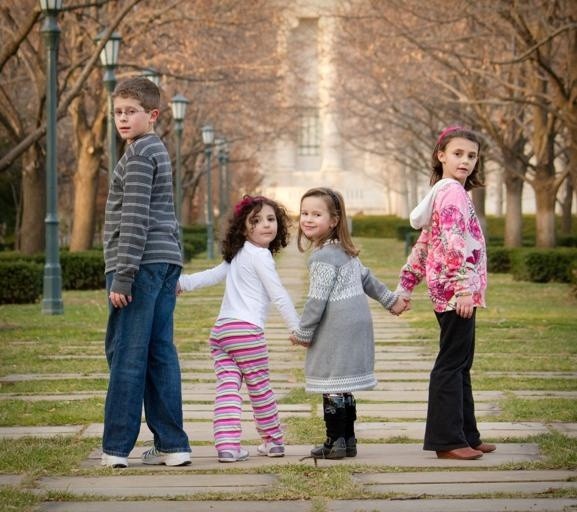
[200,122,214,258]
[141,66,160,94]
[169,90,188,264]
[38,0,66,315]
[94,24,121,188]
[219,149,231,215]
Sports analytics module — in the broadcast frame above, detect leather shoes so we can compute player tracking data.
[436,443,497,459]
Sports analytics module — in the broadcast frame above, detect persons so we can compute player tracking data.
[290,186,412,459]
[175,194,300,463]
[390,127,496,460]
[100,78,192,466]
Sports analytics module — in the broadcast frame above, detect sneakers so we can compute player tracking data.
[310,436,357,459]
[99,446,128,468]
[217,447,250,462]
[140,446,193,467]
[256,443,286,457]
